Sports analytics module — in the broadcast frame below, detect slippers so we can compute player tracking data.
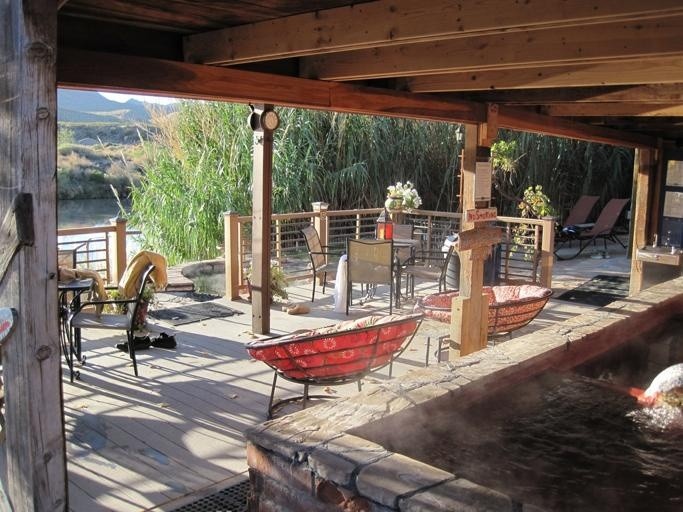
[281,302,311,316]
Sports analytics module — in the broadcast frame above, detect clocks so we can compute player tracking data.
[260,110,279,133]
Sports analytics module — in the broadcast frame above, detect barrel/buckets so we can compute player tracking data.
[441,242,498,290]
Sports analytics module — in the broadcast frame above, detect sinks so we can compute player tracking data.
[635,245,683,265]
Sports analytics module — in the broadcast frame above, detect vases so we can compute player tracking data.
[384,195,403,213]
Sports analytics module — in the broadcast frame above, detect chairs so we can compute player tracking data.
[415,282,554,364]
[246,308,426,420]
[554,194,630,261]
[298,225,454,317]
[56,248,155,385]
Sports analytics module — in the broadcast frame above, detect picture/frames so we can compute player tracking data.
[661,158,683,221]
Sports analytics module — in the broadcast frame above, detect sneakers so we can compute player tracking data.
[150,332,177,349]
[116,335,152,352]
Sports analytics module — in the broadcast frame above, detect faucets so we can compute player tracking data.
[664,230,672,248]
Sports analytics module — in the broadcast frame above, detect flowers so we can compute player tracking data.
[388,180,423,209]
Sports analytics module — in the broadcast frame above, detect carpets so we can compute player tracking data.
[555,274,630,307]
[146,302,244,327]
